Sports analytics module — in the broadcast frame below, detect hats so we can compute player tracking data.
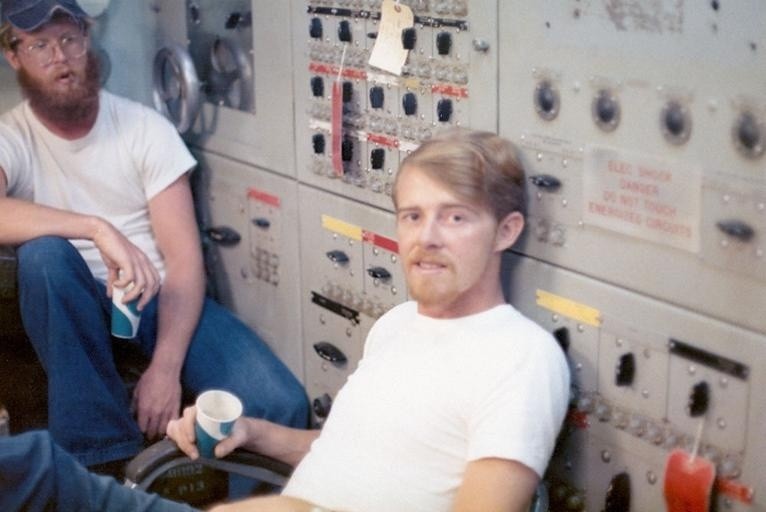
[0,0,88,31]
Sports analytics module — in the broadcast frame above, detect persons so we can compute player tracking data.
[1,0,310,466]
[0,124,570,512]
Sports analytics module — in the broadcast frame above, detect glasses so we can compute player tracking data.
[16,35,86,67]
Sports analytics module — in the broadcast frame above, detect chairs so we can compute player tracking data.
[0,152,240,309]
[118,434,551,512]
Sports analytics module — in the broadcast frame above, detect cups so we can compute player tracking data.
[110,278,142,340]
[193,390,245,460]
